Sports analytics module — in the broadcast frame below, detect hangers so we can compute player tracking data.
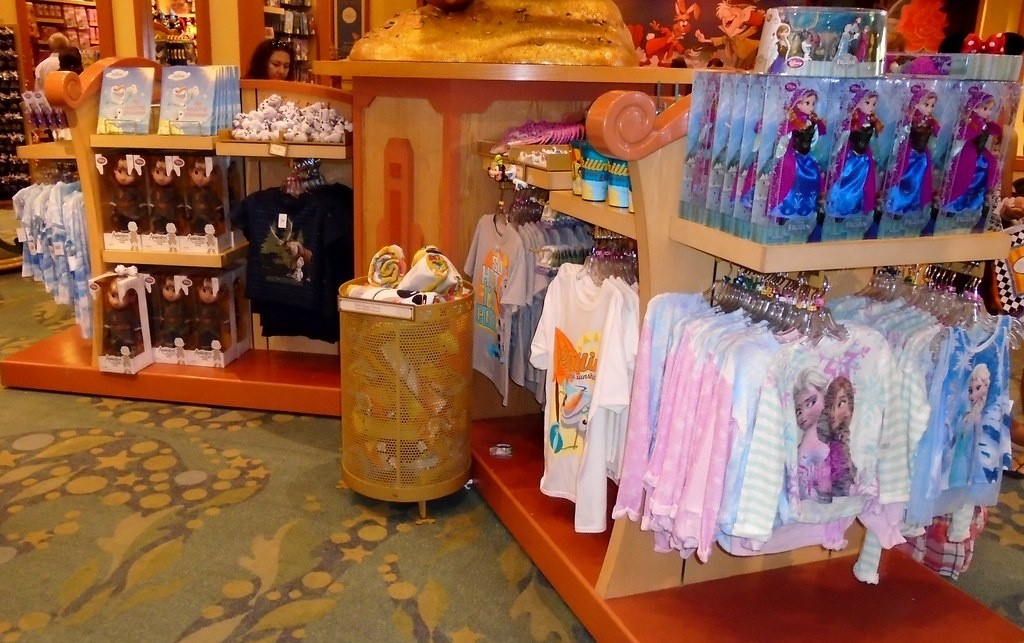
[494,182,575,228]
[702,263,847,348]
[31,167,82,192]
[576,226,639,288]
[280,158,327,198]
[853,262,1024,363]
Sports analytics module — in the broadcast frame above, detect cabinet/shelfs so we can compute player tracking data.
[88,132,249,269]
[15,0,101,182]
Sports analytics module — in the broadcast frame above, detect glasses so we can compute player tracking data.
[272,40,293,49]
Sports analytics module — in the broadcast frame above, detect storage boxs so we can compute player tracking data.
[144,269,188,365]
[223,156,248,248]
[133,154,174,253]
[173,155,232,255]
[88,271,154,375]
[181,272,237,369]
[94,150,143,251]
[227,263,253,359]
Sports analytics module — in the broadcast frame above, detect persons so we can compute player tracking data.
[241,39,296,81]
[100,276,142,357]
[34,33,69,92]
[148,154,183,233]
[158,274,191,350]
[108,157,144,232]
[192,272,231,350]
[686,78,1004,226]
[183,155,223,234]
